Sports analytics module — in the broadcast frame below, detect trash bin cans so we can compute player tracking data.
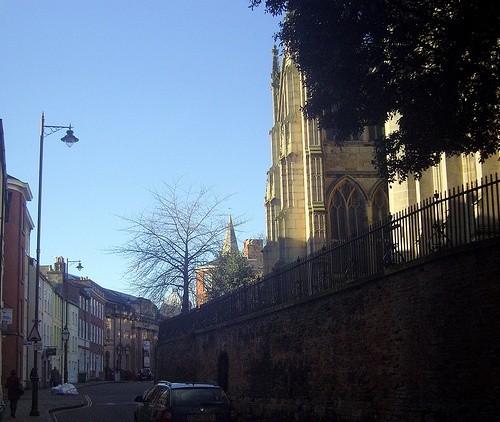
[78,372,86,382]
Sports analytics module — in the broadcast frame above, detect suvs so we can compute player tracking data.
[133,380,231,422]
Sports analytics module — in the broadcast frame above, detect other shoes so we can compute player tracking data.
[11,414,16,419]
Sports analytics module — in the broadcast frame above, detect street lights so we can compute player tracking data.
[62,258,85,384]
[27,111,79,418]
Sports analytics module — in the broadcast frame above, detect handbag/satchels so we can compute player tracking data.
[14,382,26,396]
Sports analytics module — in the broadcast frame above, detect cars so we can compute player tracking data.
[139,368,152,379]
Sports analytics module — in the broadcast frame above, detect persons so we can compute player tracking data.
[7,369,19,418]
[52,367,59,386]
